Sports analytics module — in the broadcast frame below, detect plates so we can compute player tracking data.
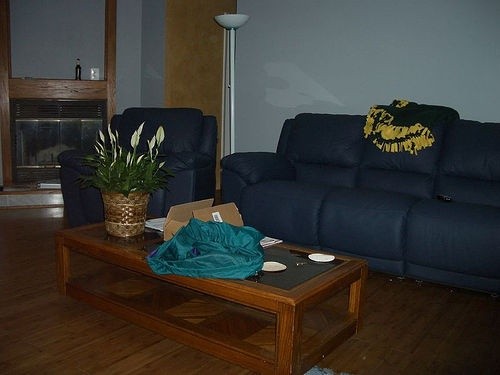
[308,253,336,262]
[261,261,288,272]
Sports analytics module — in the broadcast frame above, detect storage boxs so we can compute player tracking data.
[164,199,244,241]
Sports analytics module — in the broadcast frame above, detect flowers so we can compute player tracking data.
[72,121,177,197]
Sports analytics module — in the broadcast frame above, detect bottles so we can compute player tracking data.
[75,58,81,80]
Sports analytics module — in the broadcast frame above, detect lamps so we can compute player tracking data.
[215,14,250,154]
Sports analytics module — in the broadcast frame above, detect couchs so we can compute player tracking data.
[56,108,217,227]
[220,113,500,297]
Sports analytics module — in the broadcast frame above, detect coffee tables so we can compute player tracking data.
[55,214,369,375]
